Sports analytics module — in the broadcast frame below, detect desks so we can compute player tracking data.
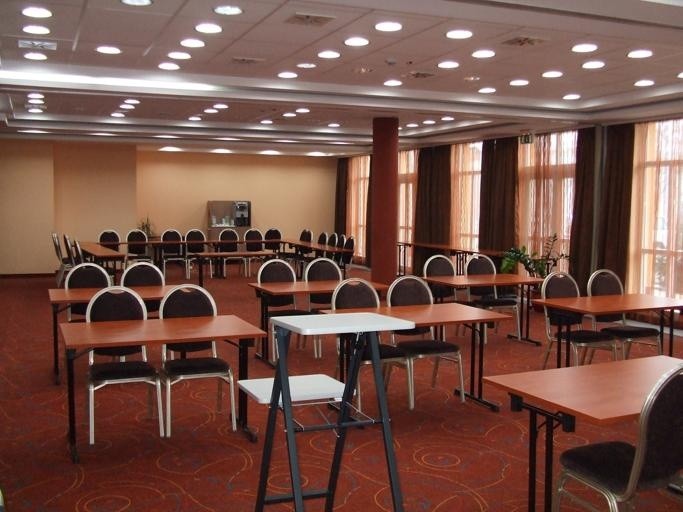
[48,284,178,386]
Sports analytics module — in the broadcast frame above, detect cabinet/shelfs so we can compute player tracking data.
[207,201,251,252]
